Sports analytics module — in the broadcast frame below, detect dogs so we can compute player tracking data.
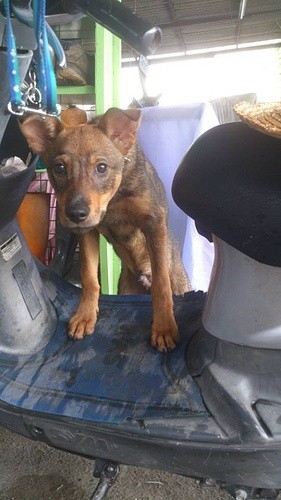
[18,108,194,356]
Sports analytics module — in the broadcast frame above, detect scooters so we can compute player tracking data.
[0,0,280,499]
